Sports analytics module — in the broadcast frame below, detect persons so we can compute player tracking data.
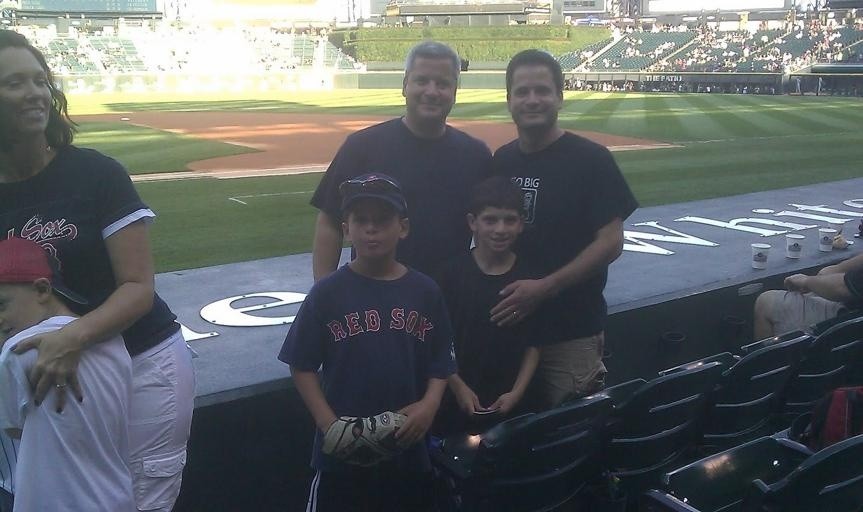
[306,41,496,288]
[275,172,456,511]
[0,29,201,510]
[752,253,862,343]
[2,20,379,78]
[419,173,560,435]
[477,47,635,408]
[549,0,862,97]
[0,239,140,510]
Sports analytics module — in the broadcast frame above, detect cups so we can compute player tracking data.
[750,242,771,270]
[784,233,806,261]
[828,220,844,240]
[817,227,837,253]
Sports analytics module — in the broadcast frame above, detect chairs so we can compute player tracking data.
[426,309,863,512]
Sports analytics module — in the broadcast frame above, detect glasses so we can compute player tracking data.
[339,180,399,195]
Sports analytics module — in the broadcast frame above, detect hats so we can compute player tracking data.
[0,238,88,306]
[342,173,409,215]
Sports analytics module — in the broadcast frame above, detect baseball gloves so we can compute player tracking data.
[320,412,411,472]
